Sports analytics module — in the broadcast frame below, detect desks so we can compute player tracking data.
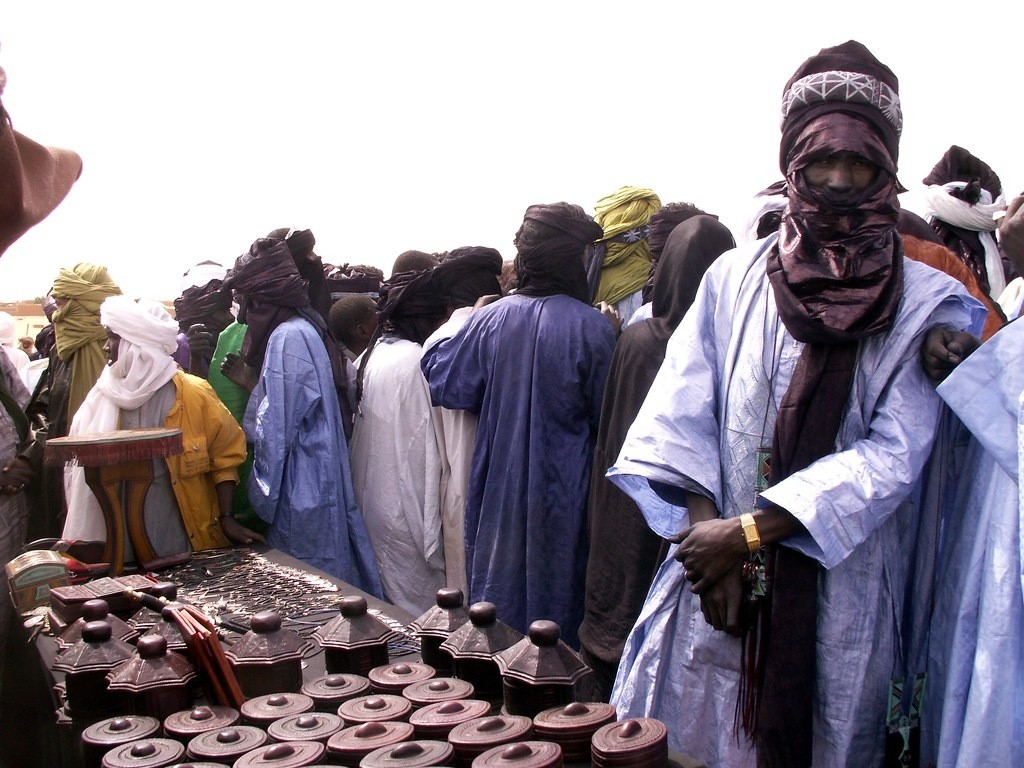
[18,535,694,767]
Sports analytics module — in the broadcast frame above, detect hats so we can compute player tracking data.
[777,40,903,178]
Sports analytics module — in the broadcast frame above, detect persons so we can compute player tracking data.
[0,146,1024,766]
[602,38,989,767]
